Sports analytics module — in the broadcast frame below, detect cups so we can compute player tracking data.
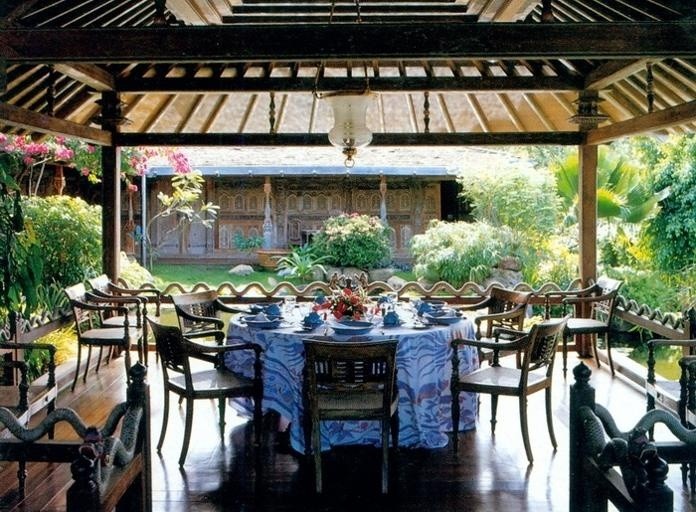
[284,295,297,306]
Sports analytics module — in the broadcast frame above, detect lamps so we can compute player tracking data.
[312,58,379,168]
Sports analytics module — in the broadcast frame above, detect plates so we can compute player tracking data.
[329,322,375,335]
[422,299,448,310]
[241,314,286,329]
[423,311,464,324]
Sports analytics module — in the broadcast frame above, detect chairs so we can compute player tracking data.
[643,339,696,504]
[1,342,59,498]
